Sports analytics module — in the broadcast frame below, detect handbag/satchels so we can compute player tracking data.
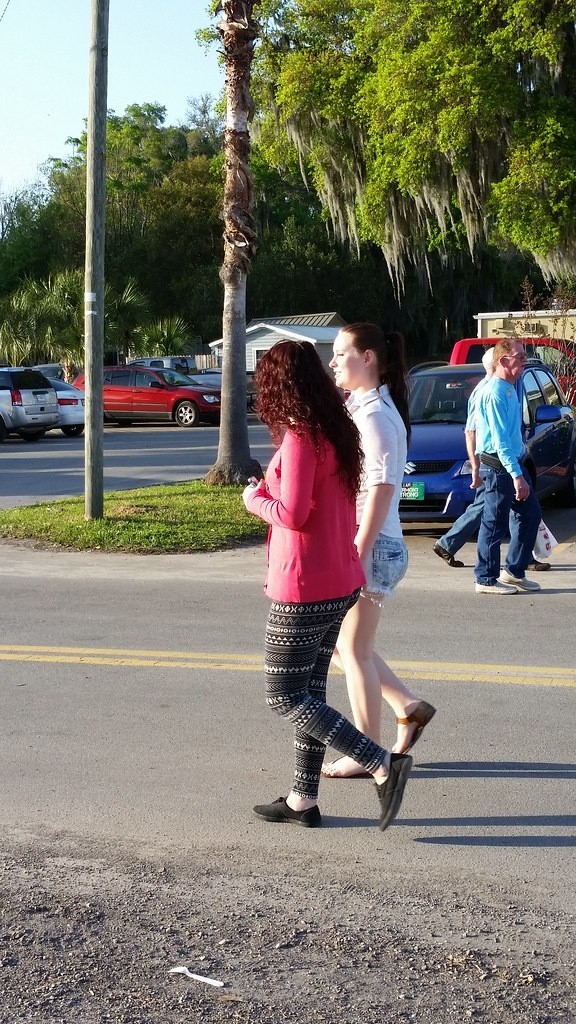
[531,518,558,563]
[441,458,476,515]
[480,452,501,470]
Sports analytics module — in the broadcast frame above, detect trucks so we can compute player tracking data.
[440,309,576,409]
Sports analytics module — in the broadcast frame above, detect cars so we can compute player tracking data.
[46,376,85,437]
[398,357,576,523]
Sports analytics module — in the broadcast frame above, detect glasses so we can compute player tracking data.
[504,351,527,358]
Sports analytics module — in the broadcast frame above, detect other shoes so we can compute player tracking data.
[431,540,464,567]
[526,558,552,571]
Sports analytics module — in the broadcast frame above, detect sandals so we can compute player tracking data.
[391,698,437,755]
[320,754,374,778]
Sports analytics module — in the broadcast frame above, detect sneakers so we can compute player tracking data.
[498,569,541,591]
[250,795,321,827]
[373,752,413,832]
[475,579,519,594]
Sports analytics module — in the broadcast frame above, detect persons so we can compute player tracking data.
[240,319,438,834]
[431,336,542,596]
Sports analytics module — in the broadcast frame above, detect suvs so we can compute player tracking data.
[70,363,221,428]
[31,363,65,381]
[0,364,62,444]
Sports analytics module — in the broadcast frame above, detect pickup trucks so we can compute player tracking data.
[124,355,257,412]
